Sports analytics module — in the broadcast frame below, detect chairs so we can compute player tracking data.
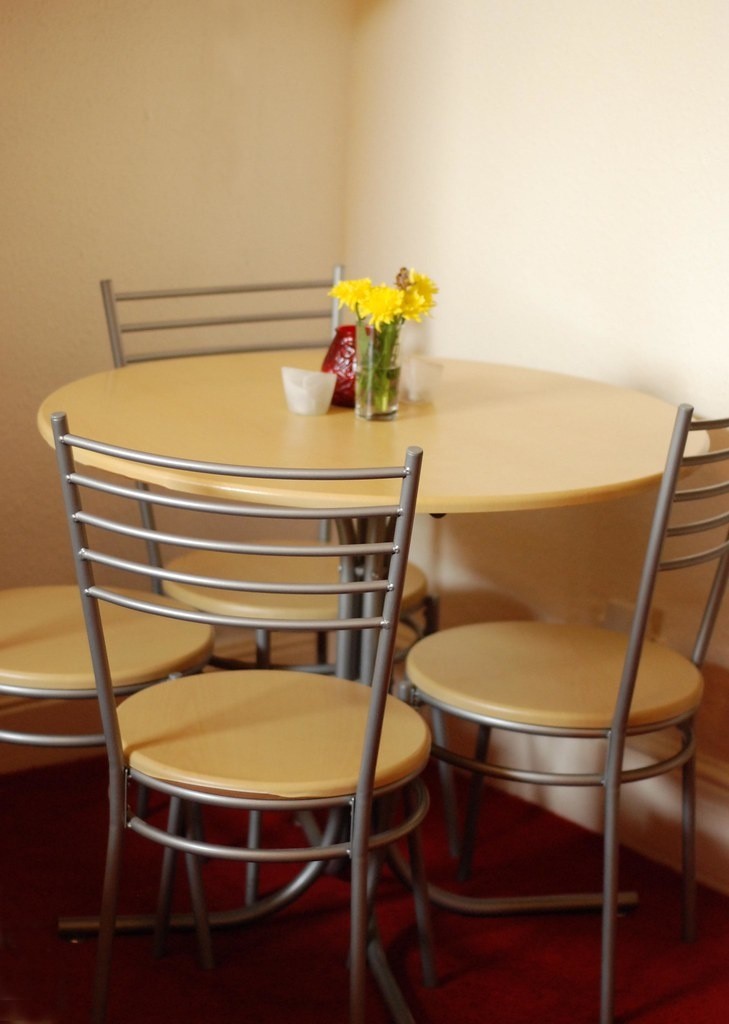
[0,261,729,1024]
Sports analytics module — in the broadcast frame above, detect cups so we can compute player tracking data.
[283,366,337,417]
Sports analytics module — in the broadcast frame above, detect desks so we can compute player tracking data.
[38,348,712,1024]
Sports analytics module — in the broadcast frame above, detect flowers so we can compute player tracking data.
[328,268,437,331]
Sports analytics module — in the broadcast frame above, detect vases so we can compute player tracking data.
[353,317,404,420]
